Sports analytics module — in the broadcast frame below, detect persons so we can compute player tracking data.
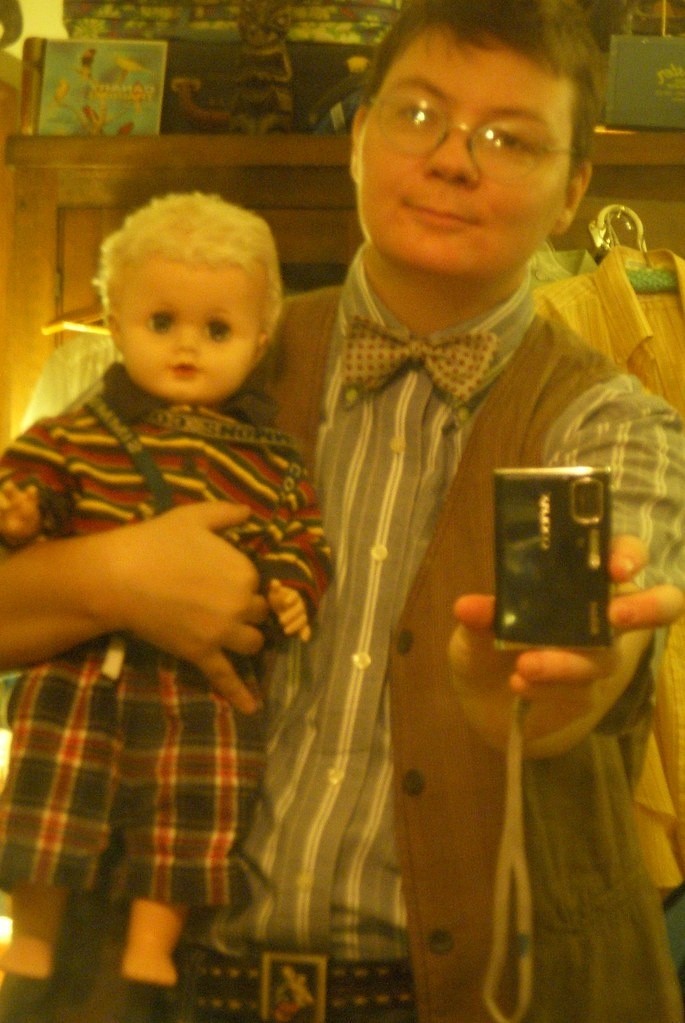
[0,0,685,1022]
[1,194,332,986]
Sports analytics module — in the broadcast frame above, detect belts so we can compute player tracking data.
[193,952,416,1023]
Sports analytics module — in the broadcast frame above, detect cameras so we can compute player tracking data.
[494,466,612,652]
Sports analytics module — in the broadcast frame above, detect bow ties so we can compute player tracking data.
[340,314,498,412]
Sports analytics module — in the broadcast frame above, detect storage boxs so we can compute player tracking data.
[19,37,168,137]
[605,34,685,131]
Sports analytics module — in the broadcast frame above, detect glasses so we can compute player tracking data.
[368,93,580,183]
